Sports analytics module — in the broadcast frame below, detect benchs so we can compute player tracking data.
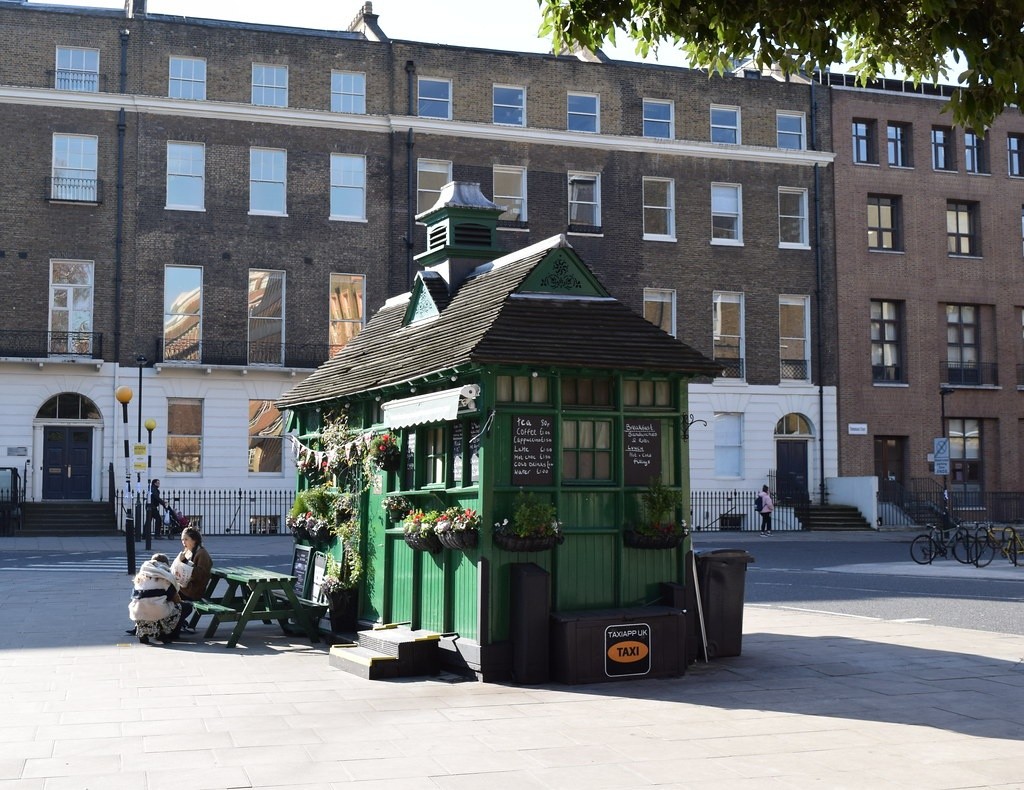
[181,600,236,621]
[273,590,329,617]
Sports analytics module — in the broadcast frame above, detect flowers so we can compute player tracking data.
[497,493,561,538]
[363,428,403,466]
[432,506,481,532]
[630,473,687,536]
[319,537,362,594]
[402,509,440,533]
[285,488,357,532]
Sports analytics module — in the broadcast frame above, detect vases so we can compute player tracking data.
[436,527,478,550]
[373,453,404,473]
[625,532,687,548]
[404,530,440,550]
[289,526,308,543]
[495,533,564,553]
[325,589,358,635]
[307,529,333,545]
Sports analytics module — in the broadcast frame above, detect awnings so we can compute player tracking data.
[382,388,476,431]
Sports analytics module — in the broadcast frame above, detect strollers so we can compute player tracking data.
[155,501,191,541]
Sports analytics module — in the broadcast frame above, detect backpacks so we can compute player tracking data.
[754,495,767,511]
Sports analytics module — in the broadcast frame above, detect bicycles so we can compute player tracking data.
[910,522,983,565]
[969,522,1024,568]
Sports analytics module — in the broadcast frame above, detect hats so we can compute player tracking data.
[152,553,169,566]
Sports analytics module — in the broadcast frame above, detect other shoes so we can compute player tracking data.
[767,531,773,536]
[158,631,172,644]
[157,632,181,641]
[155,536,165,540]
[760,533,768,537]
[139,635,150,644]
[124,626,137,635]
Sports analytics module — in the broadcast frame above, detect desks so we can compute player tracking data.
[187,566,321,647]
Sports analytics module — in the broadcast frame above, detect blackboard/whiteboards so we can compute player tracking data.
[406,430,414,486]
[452,423,462,482]
[469,421,479,482]
[290,544,314,597]
[511,414,554,485]
[624,419,662,485]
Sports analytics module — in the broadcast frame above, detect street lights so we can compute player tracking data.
[134,353,148,545]
[144,418,157,551]
[116,384,137,576]
[937,387,956,538]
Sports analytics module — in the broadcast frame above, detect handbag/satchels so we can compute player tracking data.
[170,552,194,588]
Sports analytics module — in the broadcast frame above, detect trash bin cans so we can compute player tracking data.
[695,548,755,658]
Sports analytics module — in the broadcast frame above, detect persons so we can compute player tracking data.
[758,486,773,537]
[172,508,182,519]
[129,554,193,644]
[142,479,167,539]
[125,527,213,635]
